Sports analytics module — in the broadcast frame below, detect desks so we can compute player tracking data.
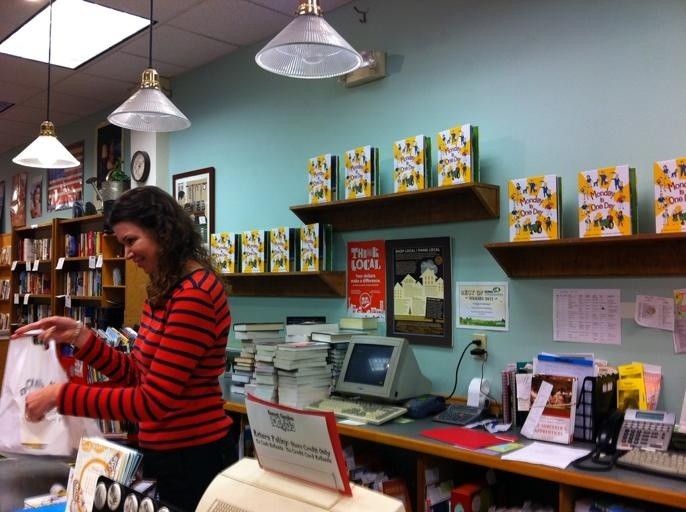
[216,373,686,511]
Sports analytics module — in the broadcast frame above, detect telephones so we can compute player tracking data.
[575,410,686,470]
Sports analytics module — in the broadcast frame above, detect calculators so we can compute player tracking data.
[433,403,487,424]
[616,409,675,454]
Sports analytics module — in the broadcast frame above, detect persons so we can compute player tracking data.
[31,184,41,219]
[8,186,241,511]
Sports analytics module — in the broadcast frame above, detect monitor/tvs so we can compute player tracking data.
[334,335,433,400]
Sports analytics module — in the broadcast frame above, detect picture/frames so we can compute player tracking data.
[172,166,214,257]
[93,118,125,210]
[385,236,454,348]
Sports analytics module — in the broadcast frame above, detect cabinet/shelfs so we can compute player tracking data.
[8,216,53,336]
[0,233,13,396]
[53,214,151,333]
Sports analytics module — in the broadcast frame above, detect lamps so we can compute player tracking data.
[0,0,159,70]
[105,0,193,135]
[10,0,81,170]
[254,1,362,80]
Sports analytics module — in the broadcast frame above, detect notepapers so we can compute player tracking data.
[89,256,95,270]
[64,296,71,308]
[14,293,19,303]
[95,255,104,268]
[10,261,17,270]
[54,257,64,269]
[24,293,30,305]
[25,262,30,272]
[56,295,65,299]
[31,260,39,272]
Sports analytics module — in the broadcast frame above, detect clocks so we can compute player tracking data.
[130,150,151,183]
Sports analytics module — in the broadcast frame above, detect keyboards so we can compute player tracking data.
[304,394,407,426]
[617,444,685,481]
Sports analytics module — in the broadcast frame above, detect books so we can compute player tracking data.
[312,318,379,392]
[508,175,563,241]
[64,268,102,297]
[0,278,10,299]
[16,300,50,326]
[344,145,379,199]
[211,232,240,273]
[437,124,480,186]
[24,438,161,511]
[64,303,104,330]
[298,224,327,271]
[239,230,270,273]
[0,312,10,334]
[578,165,639,237]
[502,363,533,426]
[19,269,51,294]
[229,316,332,407]
[267,228,298,272]
[64,229,103,257]
[0,245,12,265]
[18,236,51,261]
[394,136,432,192]
[308,154,339,203]
[654,158,685,233]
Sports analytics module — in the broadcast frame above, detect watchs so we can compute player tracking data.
[69,318,84,345]
